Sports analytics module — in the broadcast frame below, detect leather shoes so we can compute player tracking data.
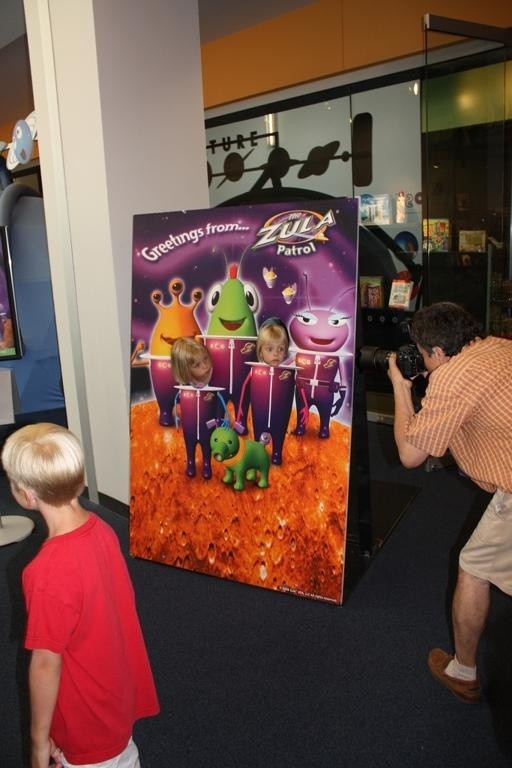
[427,648,481,705]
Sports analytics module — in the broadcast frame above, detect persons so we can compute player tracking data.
[387,302,512,705]
[253,324,287,367]
[2,422,161,768]
[167,338,212,386]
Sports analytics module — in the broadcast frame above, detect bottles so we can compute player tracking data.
[396,191,406,223]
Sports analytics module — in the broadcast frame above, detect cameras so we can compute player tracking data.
[357,344,426,378]
